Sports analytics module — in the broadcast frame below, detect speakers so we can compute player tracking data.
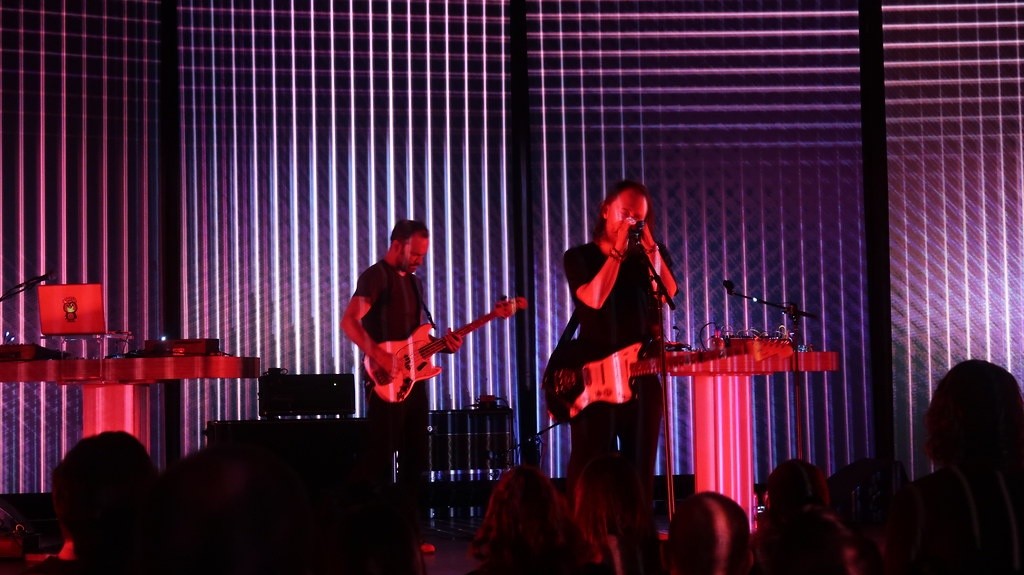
[826,455,909,532]
[206,416,370,510]
[0,492,64,554]
[257,374,355,419]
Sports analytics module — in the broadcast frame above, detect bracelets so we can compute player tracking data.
[610,248,623,260]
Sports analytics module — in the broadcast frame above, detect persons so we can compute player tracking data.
[562,179,680,517]
[340,219,463,553]
[0,356,1024,575]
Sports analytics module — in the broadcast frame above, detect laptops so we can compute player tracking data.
[37,283,132,336]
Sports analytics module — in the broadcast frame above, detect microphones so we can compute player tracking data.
[25,271,57,288]
[630,221,646,242]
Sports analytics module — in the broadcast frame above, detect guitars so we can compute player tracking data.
[360,295,528,404]
[542,336,795,420]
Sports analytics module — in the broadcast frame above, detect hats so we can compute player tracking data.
[766,461,829,501]
